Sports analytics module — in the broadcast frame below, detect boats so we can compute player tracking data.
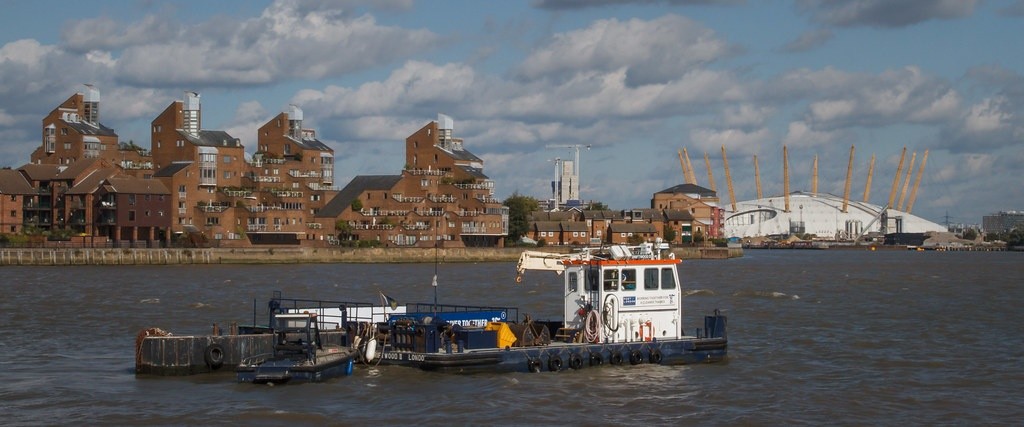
[133,215,728,387]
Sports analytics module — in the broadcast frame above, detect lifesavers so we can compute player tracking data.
[630,349,643,364]
[204,343,226,369]
[548,355,563,371]
[649,351,662,363]
[528,358,544,373]
[569,353,582,369]
[611,351,622,364]
[590,352,603,366]
[639,321,656,340]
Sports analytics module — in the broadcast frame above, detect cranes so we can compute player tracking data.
[545,143,592,177]
[547,157,561,209]
